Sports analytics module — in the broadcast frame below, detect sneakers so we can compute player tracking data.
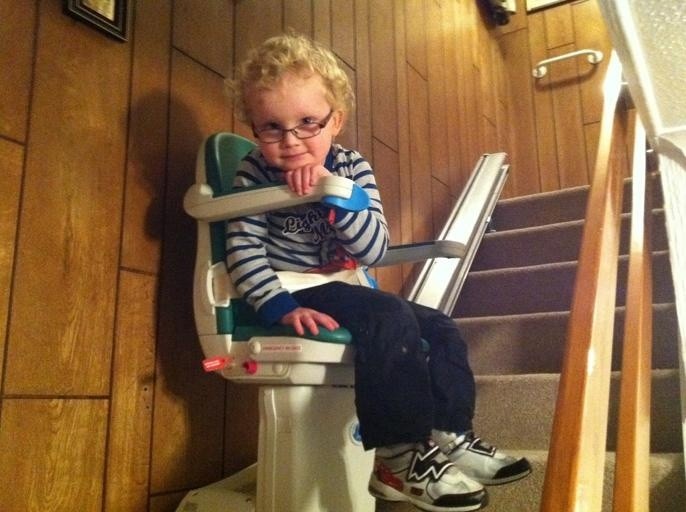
[367,439,488,512]
[431,427,533,486]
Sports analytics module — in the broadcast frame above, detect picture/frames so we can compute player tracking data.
[64,0,131,43]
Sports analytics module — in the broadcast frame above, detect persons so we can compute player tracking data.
[223,33,534,511]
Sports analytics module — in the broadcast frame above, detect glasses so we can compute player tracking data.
[249,106,336,143]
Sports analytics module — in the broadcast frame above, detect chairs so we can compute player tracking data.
[191,131,469,511]
